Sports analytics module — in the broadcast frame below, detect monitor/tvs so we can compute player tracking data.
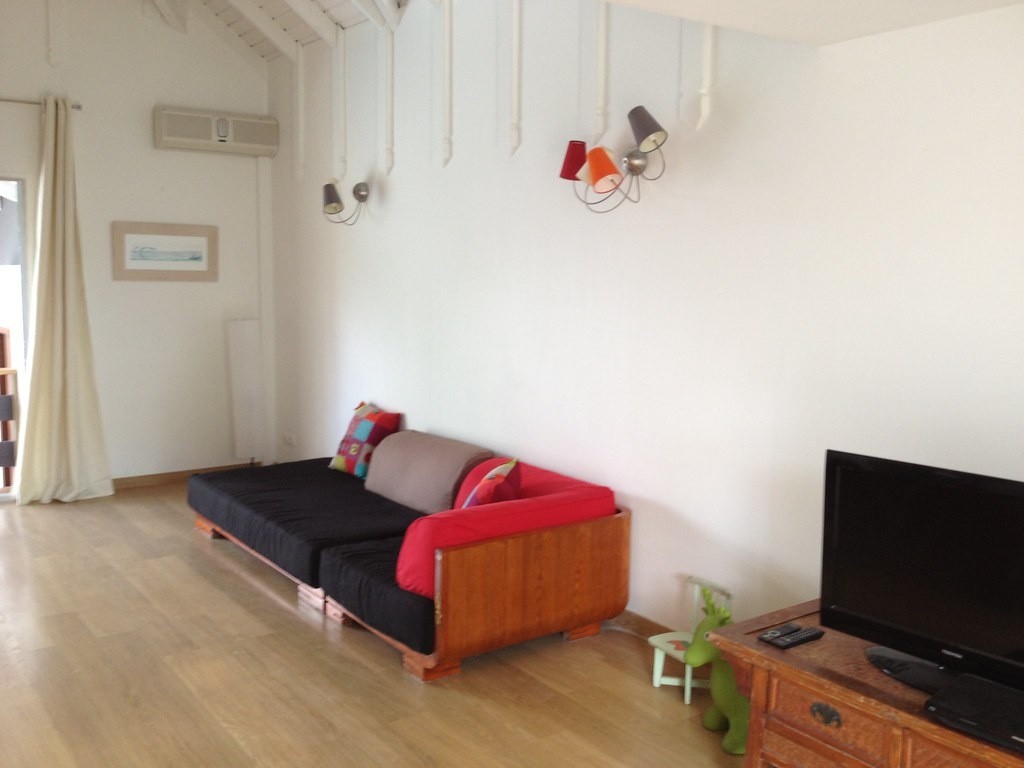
[820,449,1024,695]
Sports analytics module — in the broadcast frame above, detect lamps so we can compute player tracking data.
[322,183,369,226]
[560,105,667,213]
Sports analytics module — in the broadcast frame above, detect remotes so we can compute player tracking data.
[770,626,825,649]
[758,623,801,642]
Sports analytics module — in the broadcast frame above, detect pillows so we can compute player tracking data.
[328,401,403,479]
[363,430,495,515]
[463,455,522,512]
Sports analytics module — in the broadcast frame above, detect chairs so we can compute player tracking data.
[649,575,736,706]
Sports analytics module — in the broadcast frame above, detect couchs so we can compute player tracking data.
[186,403,631,683]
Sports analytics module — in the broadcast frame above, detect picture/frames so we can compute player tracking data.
[111,222,220,282]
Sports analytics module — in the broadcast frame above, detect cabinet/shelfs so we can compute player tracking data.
[704,598,1024,768]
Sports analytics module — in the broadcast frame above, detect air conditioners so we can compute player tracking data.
[153,107,279,159]
